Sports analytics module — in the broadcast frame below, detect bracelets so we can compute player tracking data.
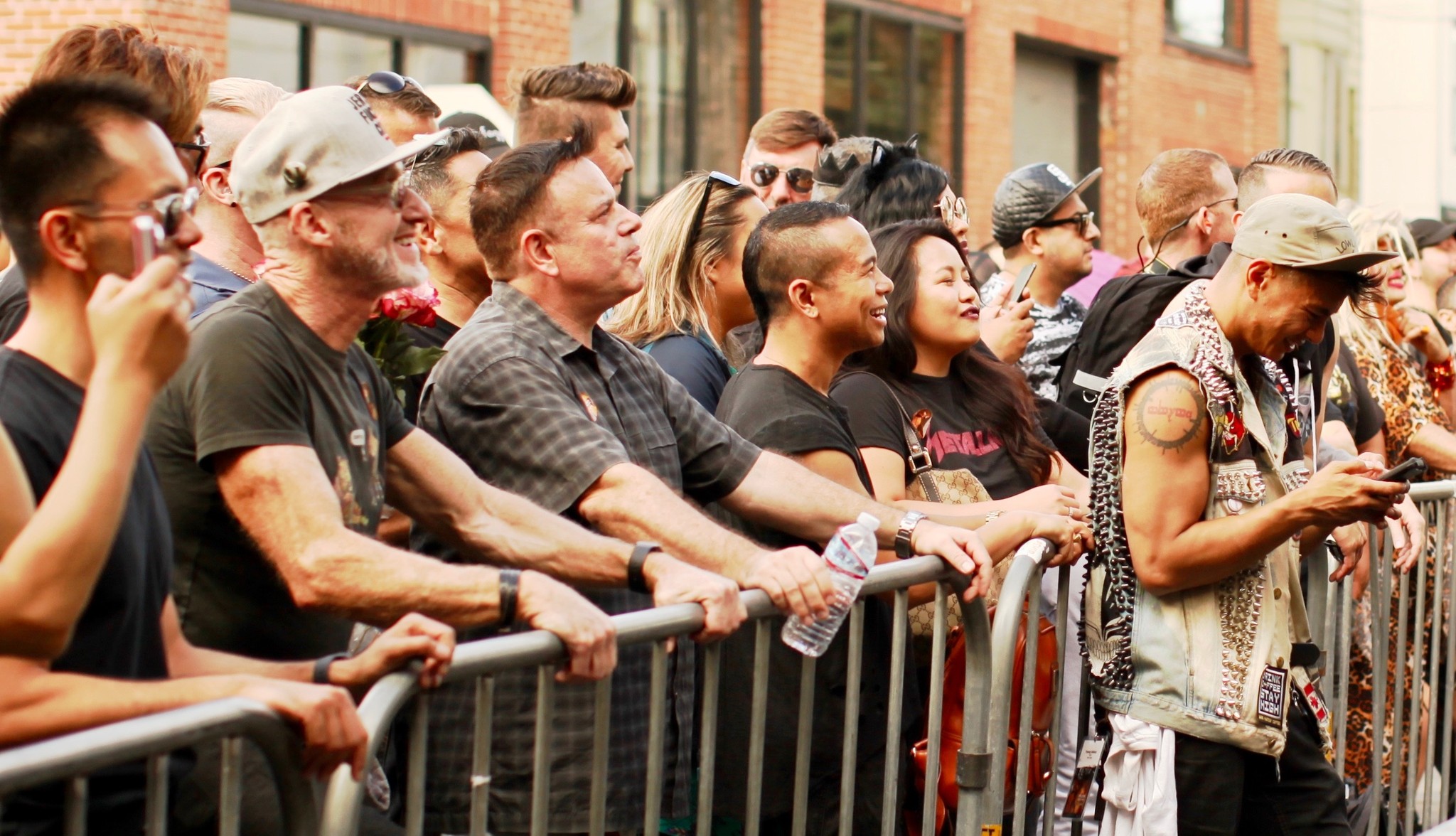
[627,542,663,595]
[313,652,351,685]
[497,566,520,635]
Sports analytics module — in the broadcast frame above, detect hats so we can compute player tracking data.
[1230,193,1399,269]
[197,77,292,168]
[1392,218,1456,261]
[439,111,512,162]
[228,87,452,224]
[991,162,1102,249]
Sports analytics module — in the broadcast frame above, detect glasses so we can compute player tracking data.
[356,71,426,94]
[934,195,970,229]
[741,160,813,193]
[66,184,200,236]
[172,141,211,175]
[680,169,741,299]
[1030,211,1095,238]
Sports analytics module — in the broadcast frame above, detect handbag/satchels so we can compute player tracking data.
[898,469,1019,635]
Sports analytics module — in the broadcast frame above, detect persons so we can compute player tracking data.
[827,148,1455,836]
[808,133,892,207]
[512,63,635,203]
[709,201,1095,836]
[742,108,831,205]
[180,66,296,326]
[343,73,442,148]
[419,123,994,836]
[1,73,458,836]
[1,24,212,348]
[140,86,747,836]
[0,188,369,836]
[600,170,774,420]
[354,126,500,552]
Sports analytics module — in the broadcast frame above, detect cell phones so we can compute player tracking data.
[996,263,1038,317]
[1371,457,1429,484]
[129,216,165,278]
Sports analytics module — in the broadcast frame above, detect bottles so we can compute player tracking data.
[780,512,880,657]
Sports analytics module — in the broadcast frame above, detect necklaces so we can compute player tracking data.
[207,257,256,287]
